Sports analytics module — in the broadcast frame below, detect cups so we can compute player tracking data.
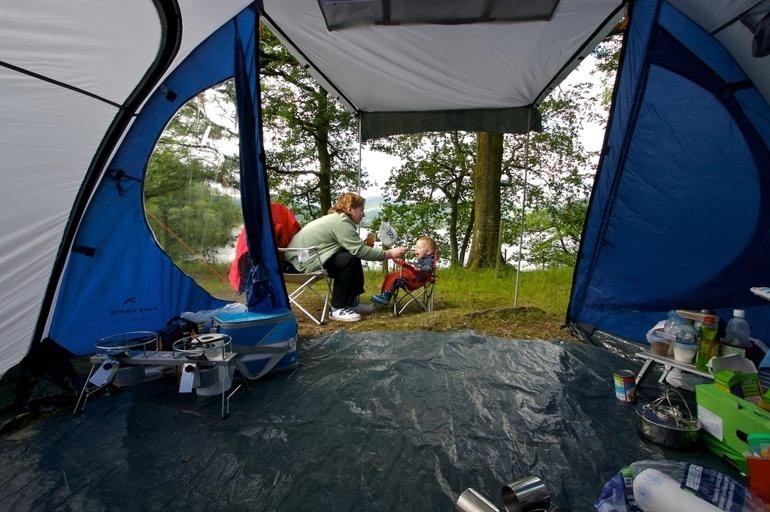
[672,342,697,363]
[500,476,550,512]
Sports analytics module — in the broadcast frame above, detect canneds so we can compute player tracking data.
[614,368,638,403]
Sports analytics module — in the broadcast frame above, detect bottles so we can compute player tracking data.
[179,322,206,352]
[720,309,751,359]
[695,315,718,373]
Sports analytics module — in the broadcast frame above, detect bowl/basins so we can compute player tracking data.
[632,403,702,446]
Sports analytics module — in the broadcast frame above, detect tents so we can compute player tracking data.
[0,0,770,511]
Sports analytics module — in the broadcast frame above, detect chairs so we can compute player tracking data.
[270,203,334,326]
[393,251,438,318]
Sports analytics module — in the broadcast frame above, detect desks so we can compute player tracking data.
[635,351,715,386]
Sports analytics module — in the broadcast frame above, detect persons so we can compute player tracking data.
[372,236,435,306]
[284,192,409,323]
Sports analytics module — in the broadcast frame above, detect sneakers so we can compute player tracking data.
[329,305,363,323]
[370,292,391,305]
[345,304,375,314]
